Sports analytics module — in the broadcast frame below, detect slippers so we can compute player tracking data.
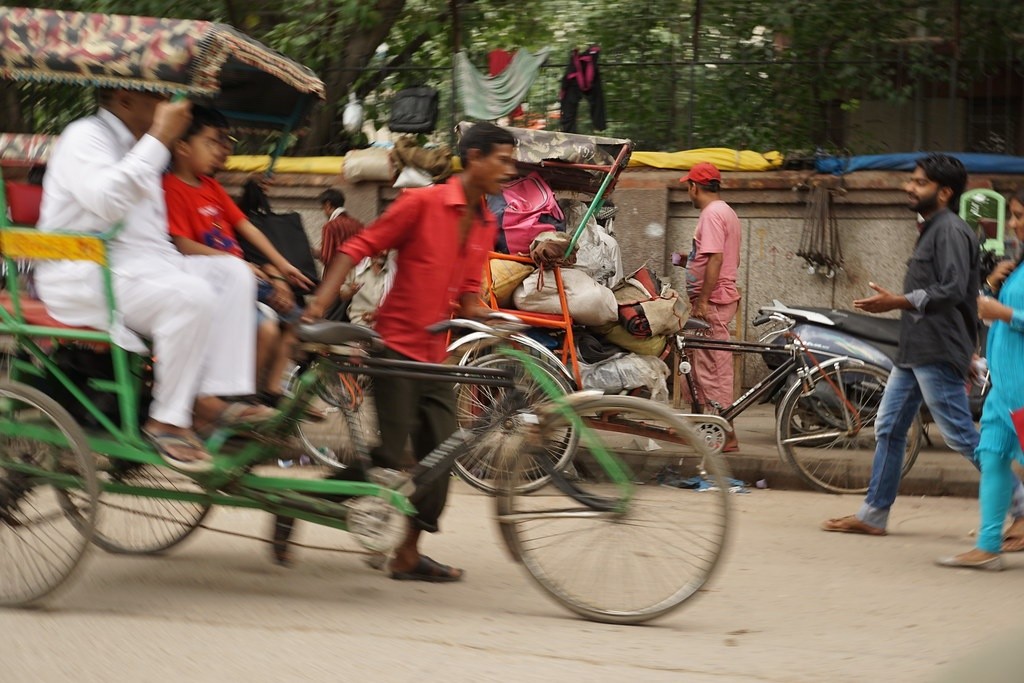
[821,515,885,536]
[141,426,214,472]
[394,554,463,582]
[1000,532,1024,553]
[196,401,282,436]
[709,442,740,452]
[271,510,296,560]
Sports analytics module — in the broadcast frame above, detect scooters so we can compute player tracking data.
[760,224,995,446]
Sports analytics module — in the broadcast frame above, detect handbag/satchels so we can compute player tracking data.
[388,85,439,132]
[485,174,567,259]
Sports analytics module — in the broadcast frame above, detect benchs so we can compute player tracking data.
[0,161,145,353]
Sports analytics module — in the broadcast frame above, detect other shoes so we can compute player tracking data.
[935,549,1007,571]
[260,385,327,422]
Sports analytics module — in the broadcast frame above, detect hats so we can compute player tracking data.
[680,161,721,186]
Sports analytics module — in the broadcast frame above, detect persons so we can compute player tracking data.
[340,250,398,330]
[35,81,282,474]
[161,102,324,424]
[934,182,1024,572]
[668,162,743,452]
[309,189,364,285]
[1,163,48,301]
[791,152,1024,552]
[270,121,520,584]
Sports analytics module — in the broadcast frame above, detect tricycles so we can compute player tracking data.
[0,6,921,626]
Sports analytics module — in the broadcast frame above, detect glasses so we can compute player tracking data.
[191,133,232,157]
